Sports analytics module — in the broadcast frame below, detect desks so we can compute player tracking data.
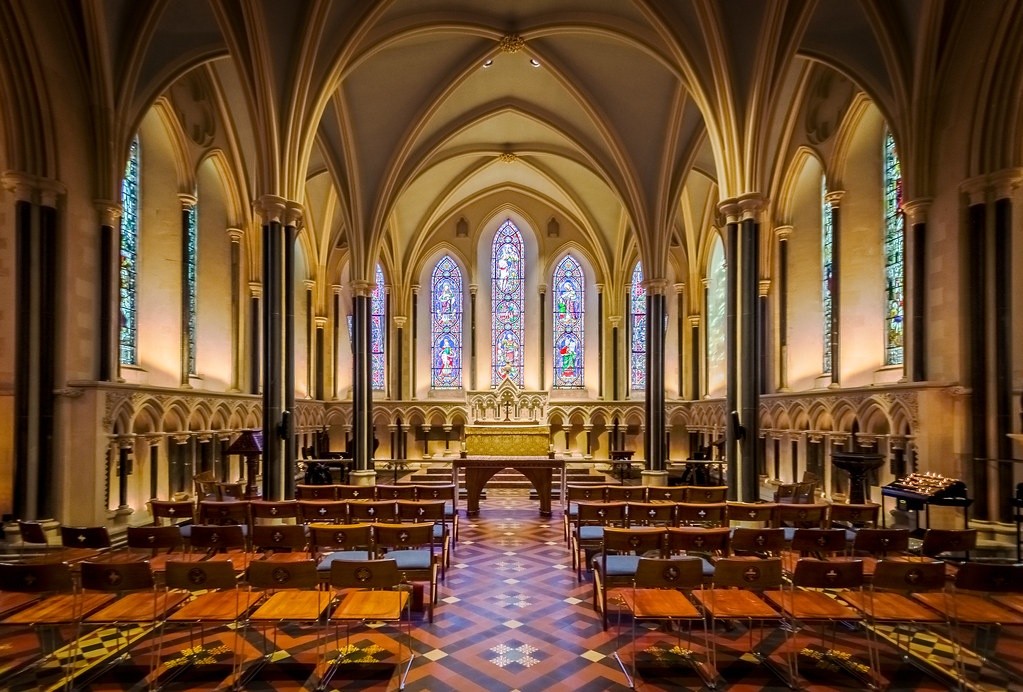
[665,459,725,486]
[295,459,353,484]
[453,451,564,519]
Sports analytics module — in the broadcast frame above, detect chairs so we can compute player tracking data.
[564,471,1023,692]
[0,469,459,692]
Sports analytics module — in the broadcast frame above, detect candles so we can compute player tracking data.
[461,442,465,450]
[551,443,554,451]
